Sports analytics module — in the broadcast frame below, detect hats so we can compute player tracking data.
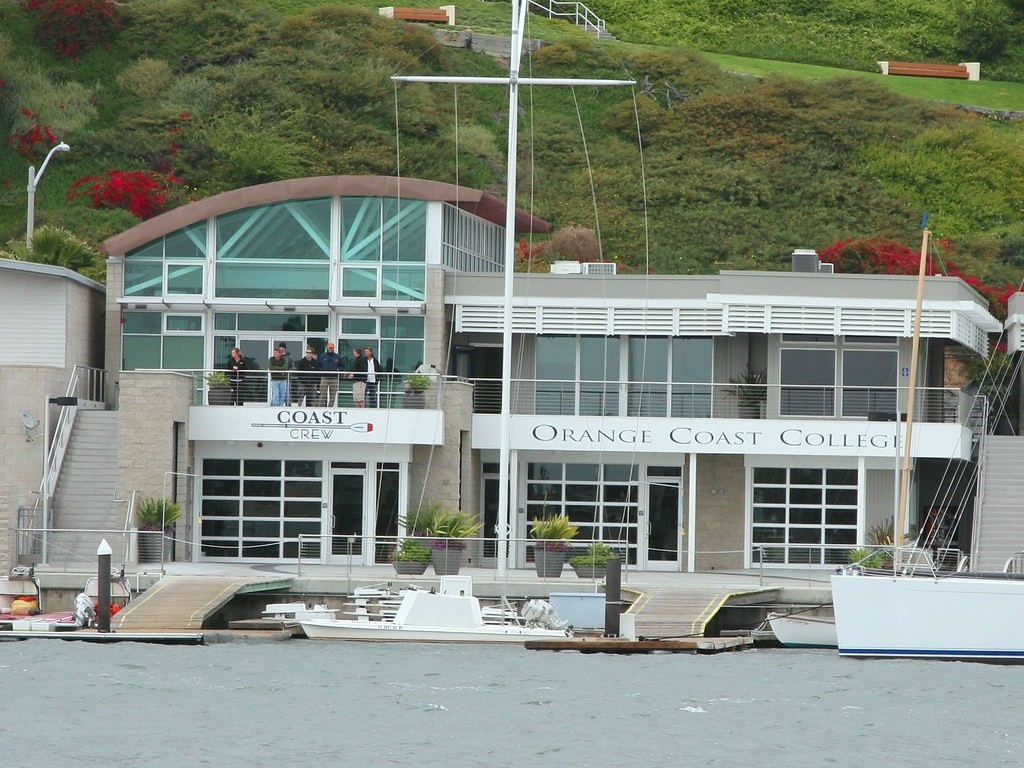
[327,343,334,348]
[279,342,286,349]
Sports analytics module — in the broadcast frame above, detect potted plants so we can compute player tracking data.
[425,507,486,575]
[204,371,232,405]
[720,364,767,419]
[835,548,883,575]
[391,538,431,574]
[402,370,430,409]
[137,495,183,562]
[867,515,894,550]
[394,499,442,549]
[569,541,618,578]
[530,512,580,577]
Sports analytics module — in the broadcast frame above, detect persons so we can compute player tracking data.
[227,341,379,408]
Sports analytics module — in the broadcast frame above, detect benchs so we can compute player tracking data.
[877,61,980,81]
[379,5,455,25]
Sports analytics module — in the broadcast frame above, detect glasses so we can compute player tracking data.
[306,352,312,354]
